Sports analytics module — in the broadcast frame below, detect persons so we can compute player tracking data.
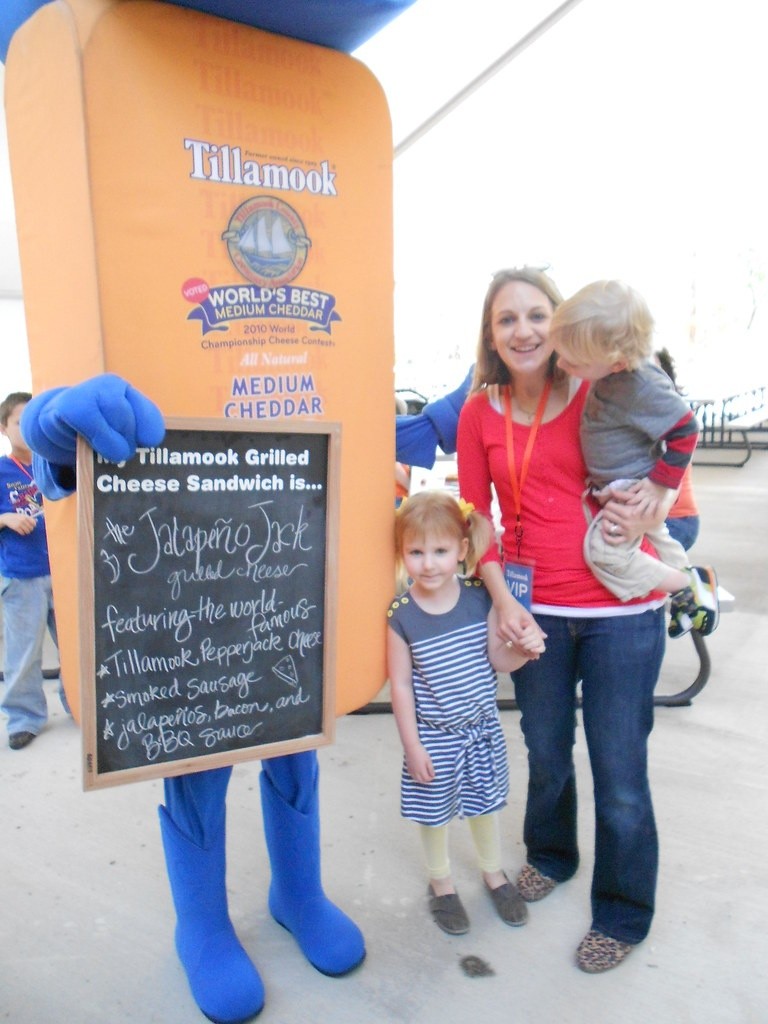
[547,280,720,638]
[385,490,546,935]
[0,391,77,751]
[456,267,670,975]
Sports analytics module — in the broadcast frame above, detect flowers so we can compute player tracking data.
[456,498,475,520]
[393,496,408,516]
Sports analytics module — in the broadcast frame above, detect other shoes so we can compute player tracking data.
[515,863,556,902]
[576,928,634,974]
[9,733,35,749]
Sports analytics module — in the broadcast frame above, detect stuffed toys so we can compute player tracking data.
[0,0,474,1024]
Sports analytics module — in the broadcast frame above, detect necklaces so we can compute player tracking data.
[512,386,536,419]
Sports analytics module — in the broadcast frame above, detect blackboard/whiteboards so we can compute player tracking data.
[76,416,343,792]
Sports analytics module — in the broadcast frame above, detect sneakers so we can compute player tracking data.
[428,883,471,934]
[669,584,697,639]
[483,869,529,927]
[682,565,720,636]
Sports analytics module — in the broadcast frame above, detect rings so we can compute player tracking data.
[609,526,618,534]
